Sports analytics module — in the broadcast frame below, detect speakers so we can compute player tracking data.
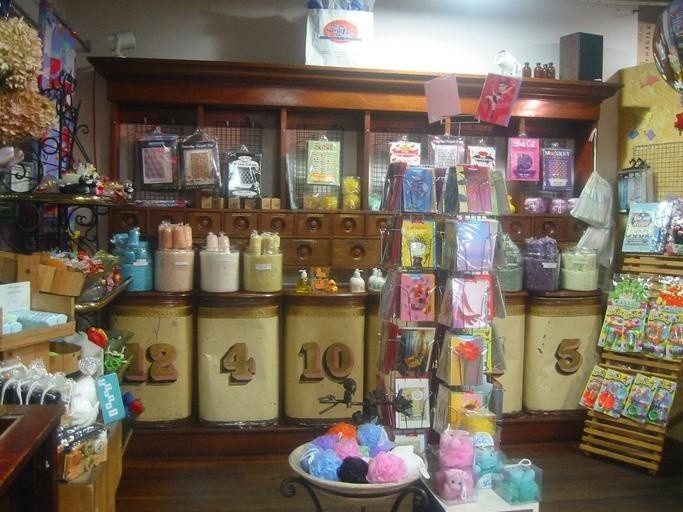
[558,33,602,81]
